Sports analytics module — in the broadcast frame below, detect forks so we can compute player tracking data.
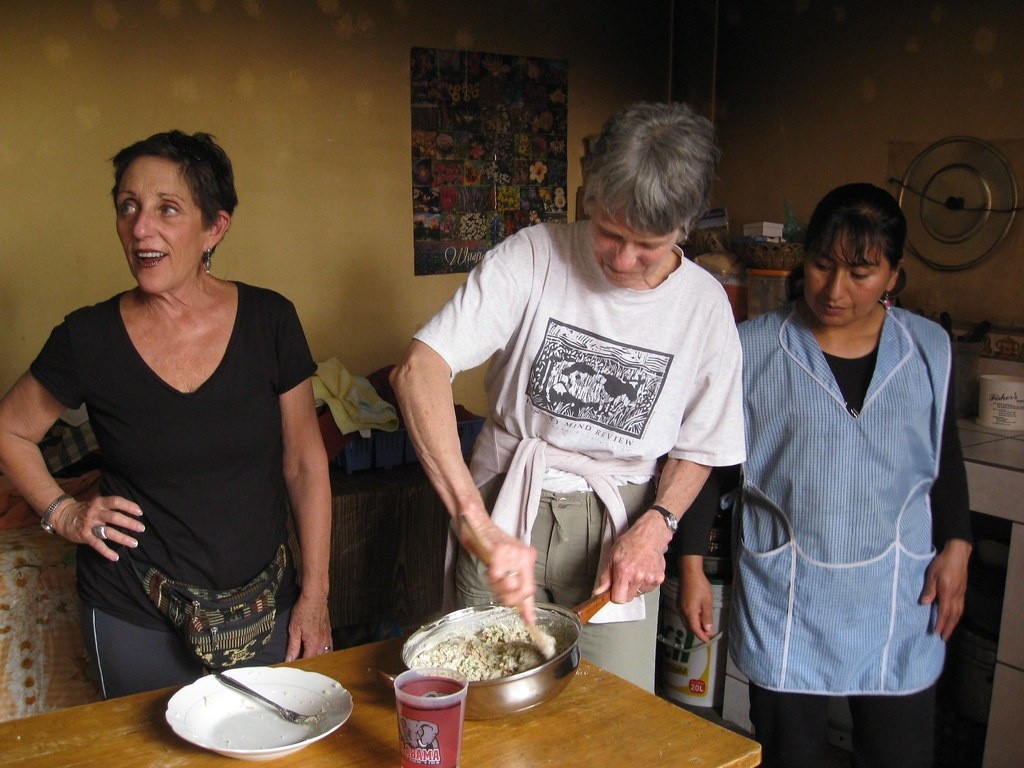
[214,674,327,726]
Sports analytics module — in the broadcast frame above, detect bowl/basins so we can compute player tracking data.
[165,666,353,762]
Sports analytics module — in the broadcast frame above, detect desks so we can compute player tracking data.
[0,632,761,768]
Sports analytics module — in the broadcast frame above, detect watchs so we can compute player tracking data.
[40,495,72,536]
[650,505,678,533]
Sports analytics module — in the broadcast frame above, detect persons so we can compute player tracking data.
[0,131,333,699]
[676,183,975,768]
[390,101,747,694]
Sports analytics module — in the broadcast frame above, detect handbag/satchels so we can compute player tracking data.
[125,540,288,669]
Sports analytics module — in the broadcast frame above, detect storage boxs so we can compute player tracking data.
[741,220,784,241]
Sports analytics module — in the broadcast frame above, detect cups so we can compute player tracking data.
[975,374,1024,431]
[745,268,791,320]
[394,668,469,768]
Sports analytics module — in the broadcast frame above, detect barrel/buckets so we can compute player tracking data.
[658,571,732,708]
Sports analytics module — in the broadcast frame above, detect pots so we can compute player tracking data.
[401,590,610,722]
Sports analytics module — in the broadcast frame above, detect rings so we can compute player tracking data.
[324,646,331,650]
[638,590,645,594]
[506,570,520,577]
[93,526,107,539]
